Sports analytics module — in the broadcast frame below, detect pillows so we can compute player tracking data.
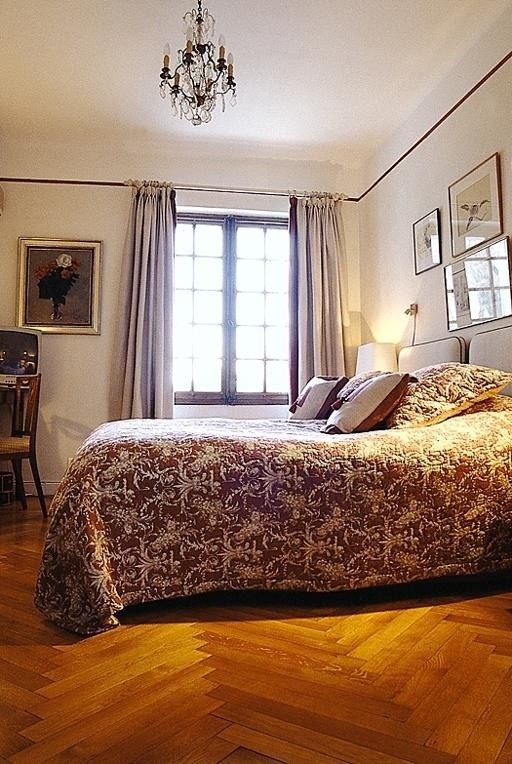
[292,363,512,434]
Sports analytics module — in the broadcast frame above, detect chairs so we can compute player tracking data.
[0,373,49,521]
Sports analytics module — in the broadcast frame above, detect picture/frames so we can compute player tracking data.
[412,152,512,332]
[15,237,103,336]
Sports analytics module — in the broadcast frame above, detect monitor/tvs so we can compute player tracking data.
[0,326,42,389]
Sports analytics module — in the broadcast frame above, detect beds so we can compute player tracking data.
[71,324,512,632]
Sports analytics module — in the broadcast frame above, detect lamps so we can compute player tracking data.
[355,342,399,377]
[158,1,238,127]
